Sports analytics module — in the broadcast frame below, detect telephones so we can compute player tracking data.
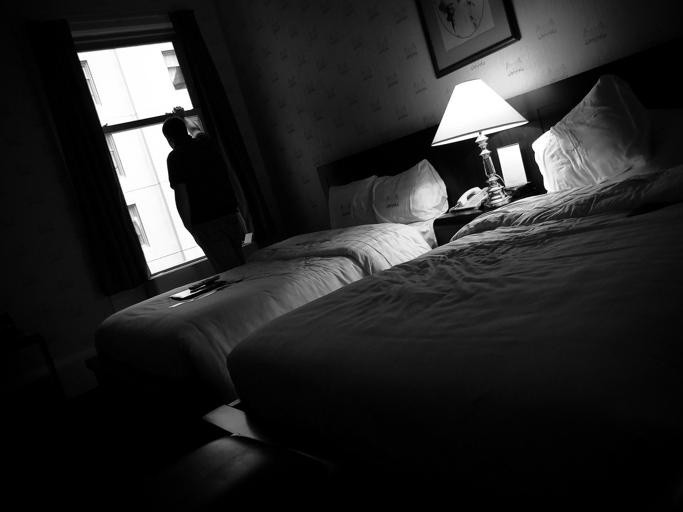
[452,187,488,210]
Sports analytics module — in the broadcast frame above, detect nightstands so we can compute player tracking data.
[434,190,536,247]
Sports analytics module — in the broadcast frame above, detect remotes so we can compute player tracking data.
[188,274,220,289]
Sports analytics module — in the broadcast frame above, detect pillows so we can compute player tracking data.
[529,59,652,198]
[327,160,448,224]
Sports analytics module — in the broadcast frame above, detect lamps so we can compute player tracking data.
[430,93,530,198]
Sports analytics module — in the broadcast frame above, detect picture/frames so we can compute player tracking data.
[413,2,521,80]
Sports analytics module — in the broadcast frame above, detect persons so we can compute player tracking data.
[160,106,247,275]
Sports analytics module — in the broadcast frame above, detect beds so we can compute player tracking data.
[229,43,681,511]
[96,122,454,401]
